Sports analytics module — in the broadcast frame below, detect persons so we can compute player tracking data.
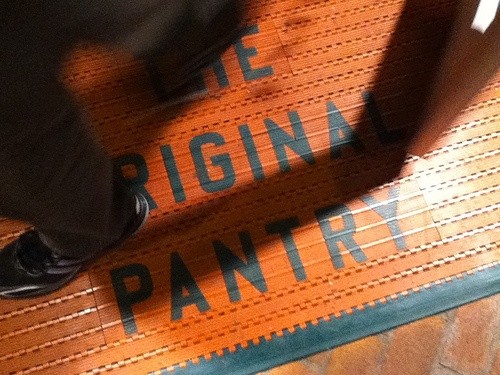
[0,1,249,301]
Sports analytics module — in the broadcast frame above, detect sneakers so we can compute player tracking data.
[0,190,150,301]
